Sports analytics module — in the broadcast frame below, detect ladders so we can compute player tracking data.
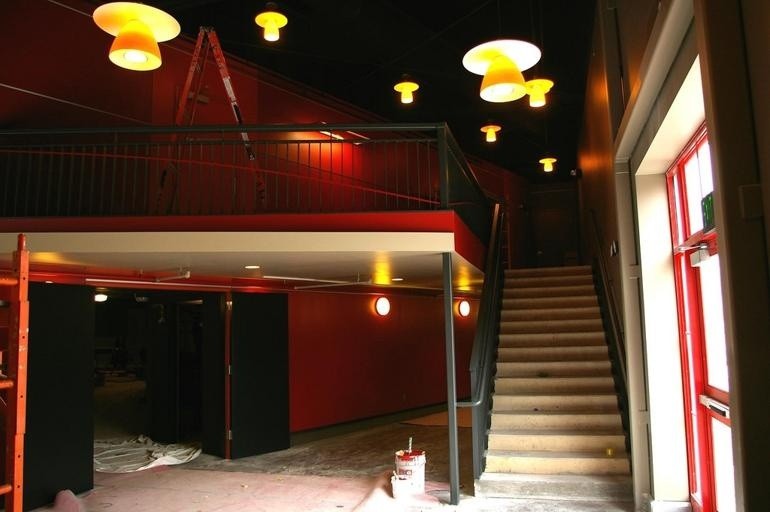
[152,25,273,216]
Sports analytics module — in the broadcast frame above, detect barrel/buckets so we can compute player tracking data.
[390,470,409,499]
[395,449,426,495]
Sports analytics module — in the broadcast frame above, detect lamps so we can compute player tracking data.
[478,115,502,144]
[94,1,181,74]
[371,297,471,320]
[251,3,289,44]
[526,70,553,111]
[392,71,421,107]
[536,150,556,171]
[459,30,541,104]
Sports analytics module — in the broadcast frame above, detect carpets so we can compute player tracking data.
[23,461,464,512]
[400,405,473,429]
